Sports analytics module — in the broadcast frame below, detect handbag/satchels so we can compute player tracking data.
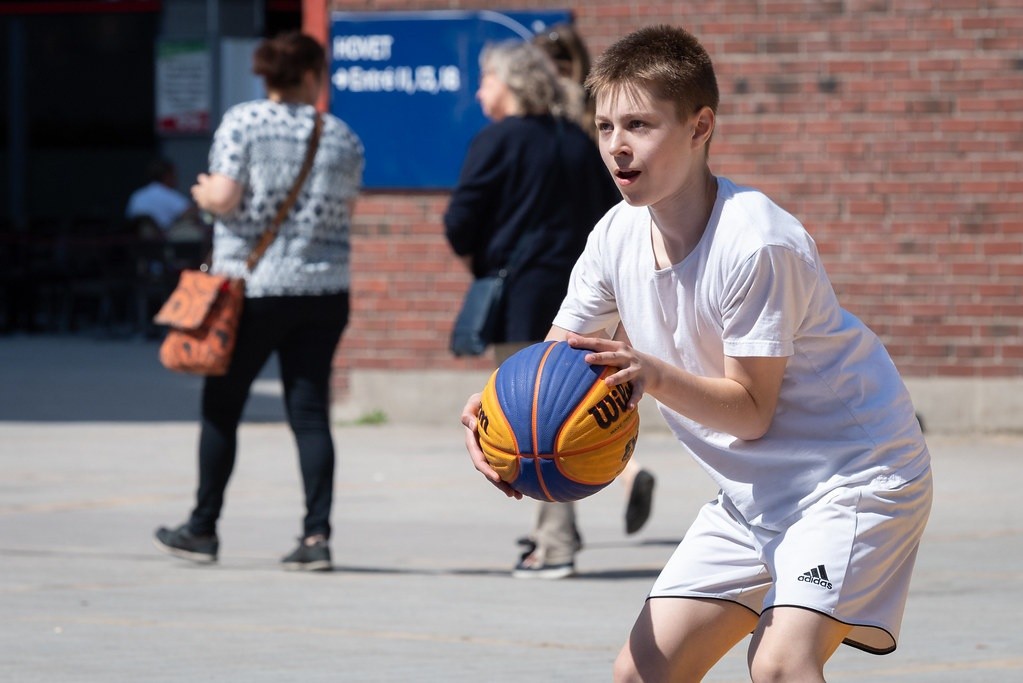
[449,275,504,357]
[153,270,246,374]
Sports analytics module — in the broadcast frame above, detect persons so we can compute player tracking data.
[461,27,934,683]
[151,31,366,571]
[444,23,653,581]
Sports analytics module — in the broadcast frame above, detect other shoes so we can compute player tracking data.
[624,469,655,534]
[512,556,574,578]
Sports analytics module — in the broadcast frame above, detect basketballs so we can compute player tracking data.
[475,341,641,504]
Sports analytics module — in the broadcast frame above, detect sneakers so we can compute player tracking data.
[280,539,332,570]
[153,522,219,563]
